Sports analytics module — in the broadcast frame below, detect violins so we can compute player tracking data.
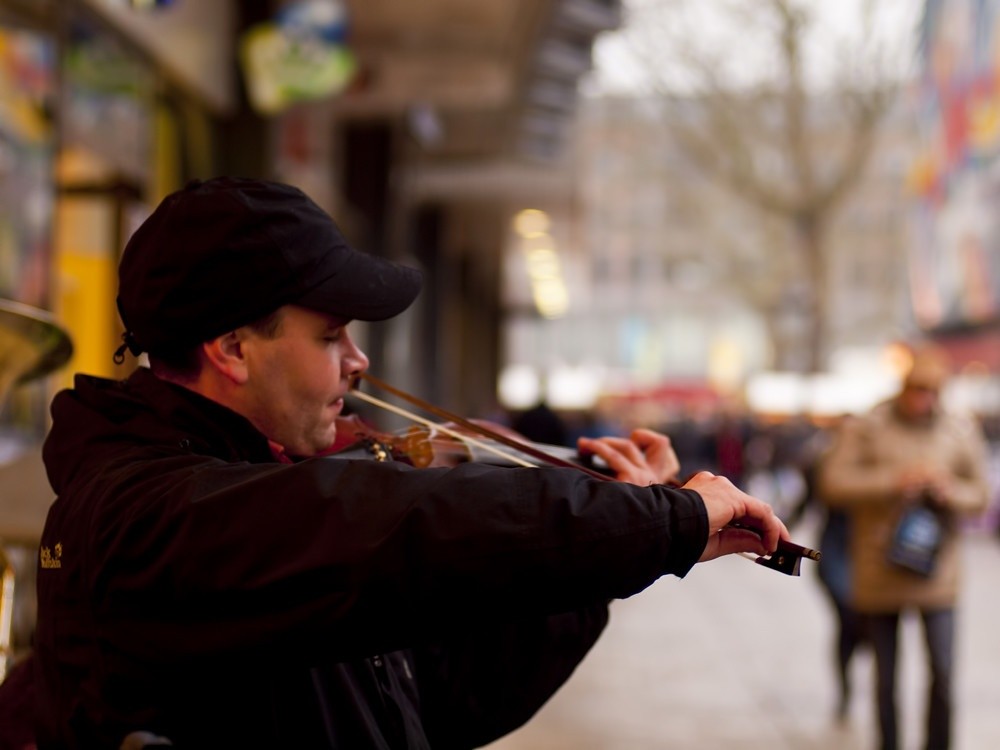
[264,408,826,578]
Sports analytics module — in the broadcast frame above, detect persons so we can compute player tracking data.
[783,413,873,726]
[0,177,790,750]
[811,353,990,750]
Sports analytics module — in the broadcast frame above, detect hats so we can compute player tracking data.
[906,358,941,395]
[116,179,424,359]
